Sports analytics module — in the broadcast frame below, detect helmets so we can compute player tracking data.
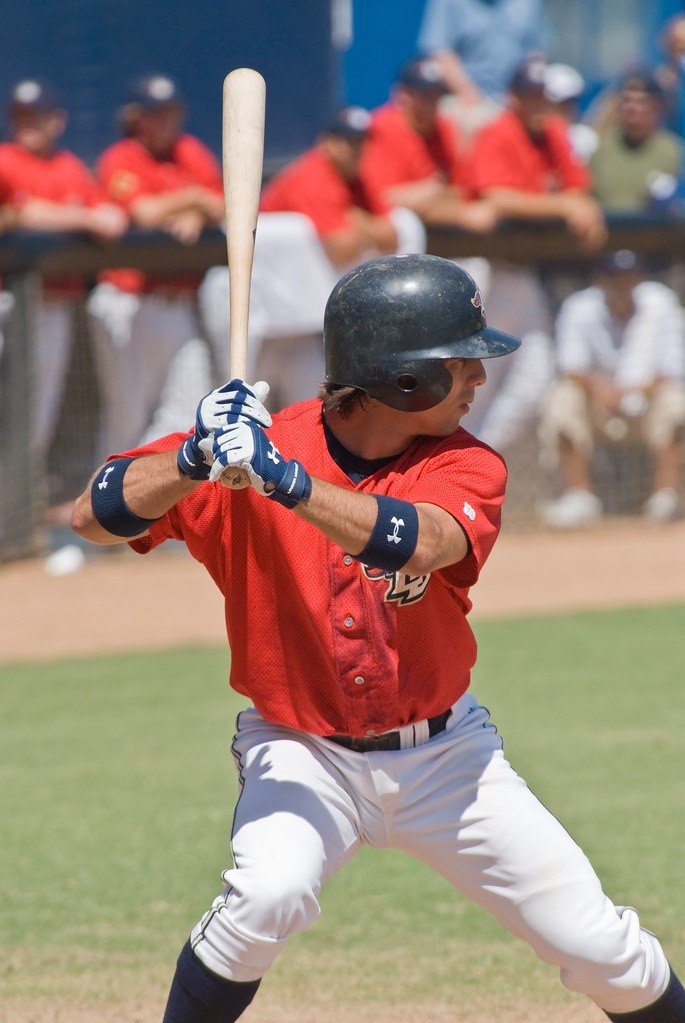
[324,253,521,411]
[512,60,546,94]
[325,104,375,140]
[13,78,63,113]
[401,63,453,94]
[599,248,640,275]
[123,75,181,109]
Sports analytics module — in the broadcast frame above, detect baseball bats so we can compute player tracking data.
[216,65,269,494]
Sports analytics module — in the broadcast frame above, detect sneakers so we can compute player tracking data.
[545,489,601,527]
[642,491,678,520]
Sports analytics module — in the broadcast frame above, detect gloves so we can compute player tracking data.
[177,378,272,481]
[196,421,312,509]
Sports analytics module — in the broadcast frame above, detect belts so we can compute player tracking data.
[323,709,452,752]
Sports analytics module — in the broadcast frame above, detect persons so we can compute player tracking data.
[0,17,685,559]
[69,255,684,1023]
